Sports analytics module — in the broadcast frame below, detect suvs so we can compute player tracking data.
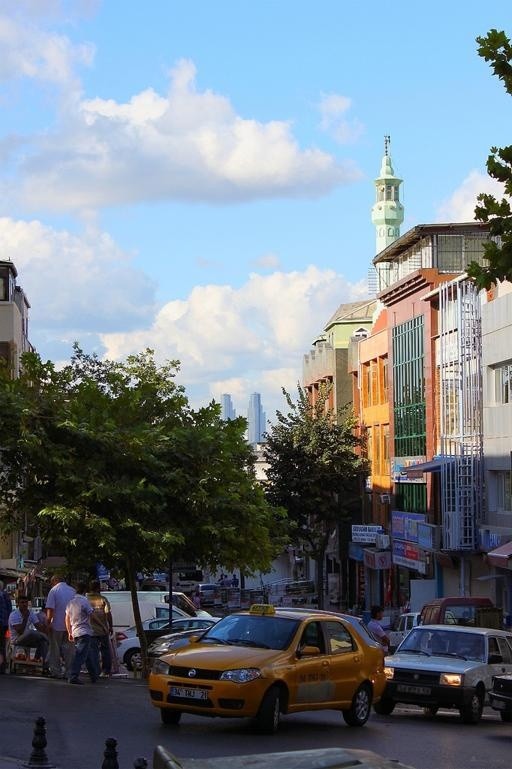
[147,606,389,667]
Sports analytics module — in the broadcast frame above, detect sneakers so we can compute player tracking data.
[41,667,113,685]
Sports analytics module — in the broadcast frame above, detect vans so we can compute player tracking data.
[419,596,494,627]
[100,590,212,636]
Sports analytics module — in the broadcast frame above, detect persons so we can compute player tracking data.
[0,571,118,685]
[367,605,390,651]
[215,573,240,588]
[138,570,143,587]
[461,640,491,662]
[193,590,201,609]
[421,634,443,655]
[145,572,150,577]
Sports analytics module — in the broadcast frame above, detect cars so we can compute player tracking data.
[386,612,422,647]
[373,625,512,725]
[147,603,387,733]
[174,578,319,609]
[487,674,512,722]
[113,617,222,672]
